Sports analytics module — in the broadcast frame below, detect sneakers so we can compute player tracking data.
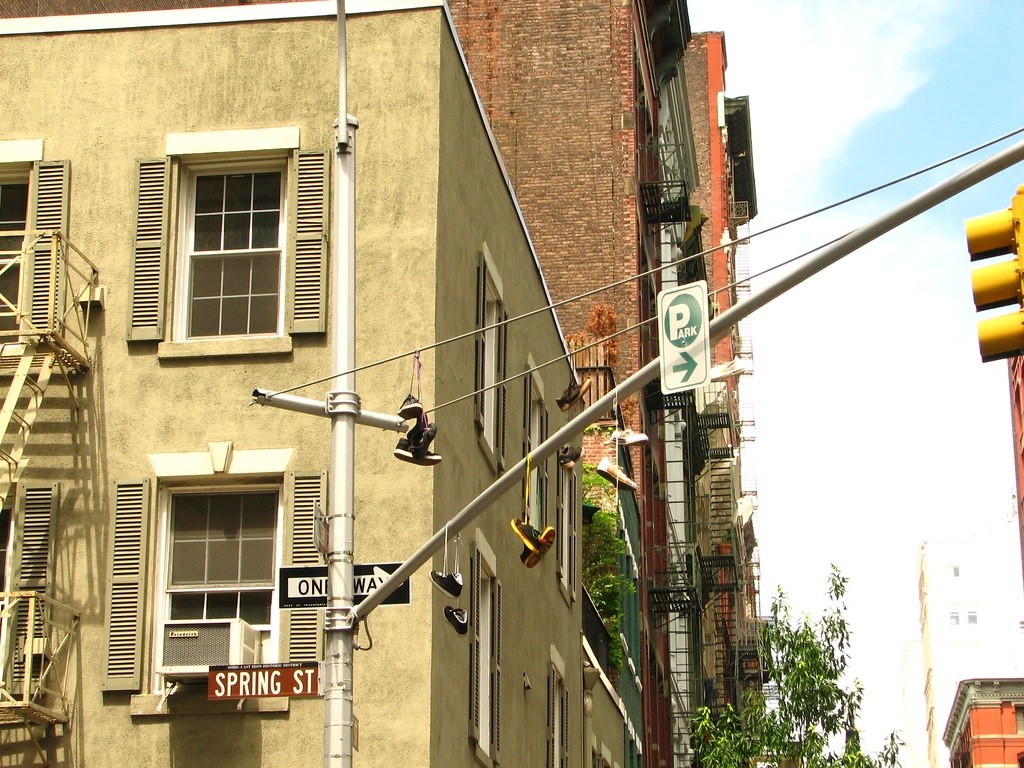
[597,457,637,490]
[511,518,546,554]
[394,438,442,466]
[520,526,555,568]
[407,416,437,462]
[555,380,591,412]
[610,430,648,446]
[559,446,583,470]
[397,395,424,419]
[429,570,463,598]
[443,606,468,634]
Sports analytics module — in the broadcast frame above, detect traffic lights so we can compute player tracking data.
[964,185,1024,363]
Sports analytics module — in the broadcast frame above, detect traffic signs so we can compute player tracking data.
[657,281,712,396]
[278,561,411,611]
